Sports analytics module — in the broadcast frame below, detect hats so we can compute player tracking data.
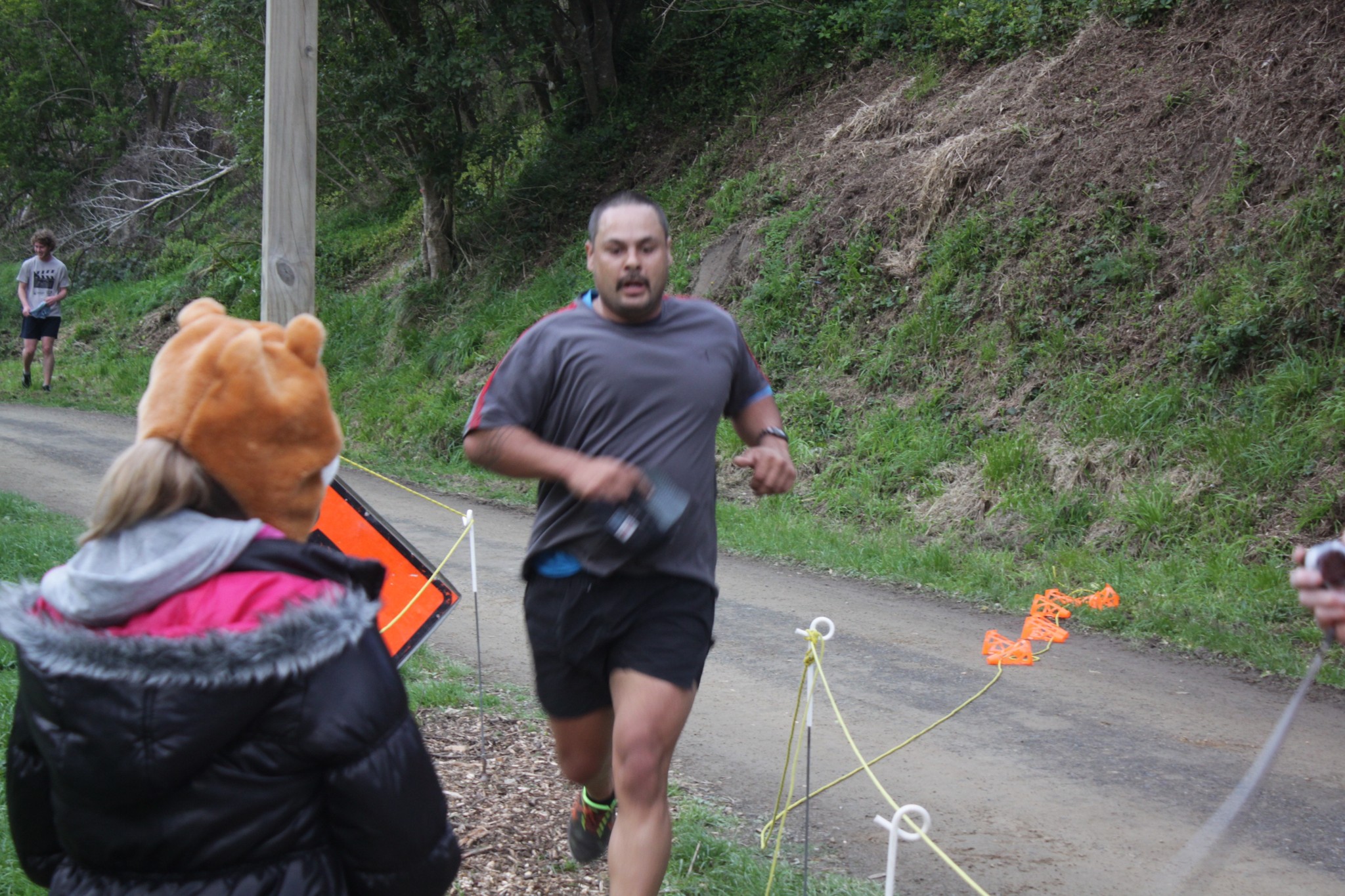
[136,296,345,544]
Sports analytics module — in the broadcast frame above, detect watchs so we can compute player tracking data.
[754,428,788,447]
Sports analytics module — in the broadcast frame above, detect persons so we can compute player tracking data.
[0,295,460,896]
[17,228,71,391]
[462,193,796,896]
[1290,529,1345,644]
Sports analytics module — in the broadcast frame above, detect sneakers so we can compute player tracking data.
[567,785,617,864]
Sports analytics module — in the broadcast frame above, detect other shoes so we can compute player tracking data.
[41,385,51,392]
[22,373,32,389]
